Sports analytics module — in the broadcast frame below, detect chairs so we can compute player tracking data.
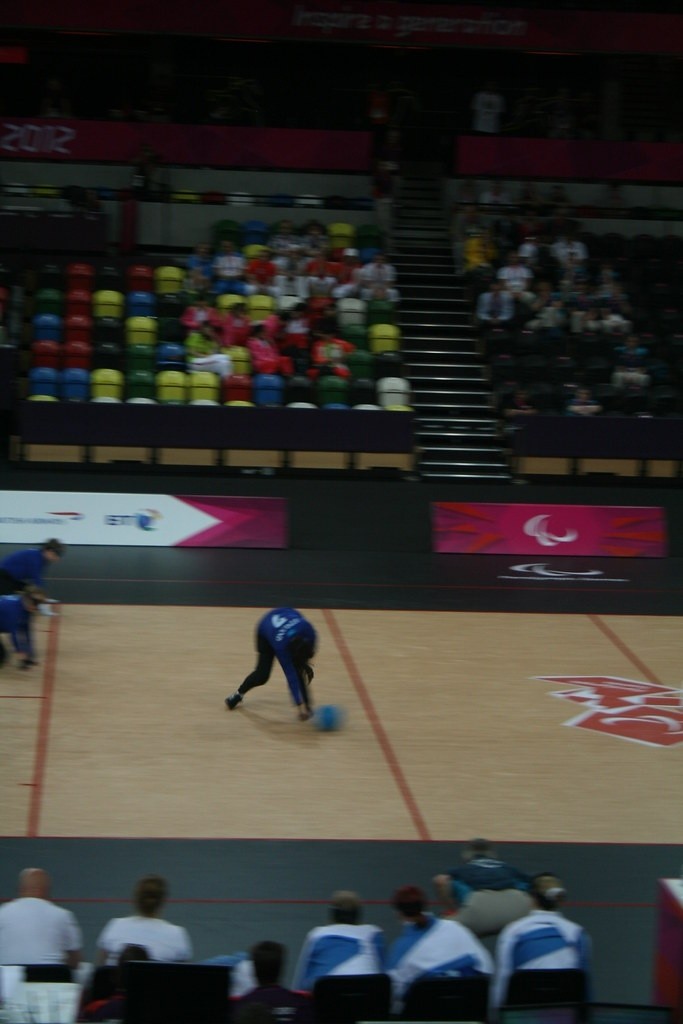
[453,200,683,483]
[0,951,676,1024]
[0,182,413,476]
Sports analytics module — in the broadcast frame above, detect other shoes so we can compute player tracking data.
[224,691,243,711]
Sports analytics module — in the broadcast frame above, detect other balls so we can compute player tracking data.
[314,704,342,732]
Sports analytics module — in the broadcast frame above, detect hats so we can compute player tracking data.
[527,873,565,909]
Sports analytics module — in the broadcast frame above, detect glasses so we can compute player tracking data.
[28,597,42,607]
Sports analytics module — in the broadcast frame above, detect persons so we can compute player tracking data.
[89,872,195,978]
[0,584,46,670]
[225,939,310,1024]
[377,883,496,1017]
[223,606,319,722]
[0,868,85,976]
[489,869,592,1021]
[430,834,536,938]
[0,537,64,621]
[290,890,385,1010]
[0,43,663,439]
